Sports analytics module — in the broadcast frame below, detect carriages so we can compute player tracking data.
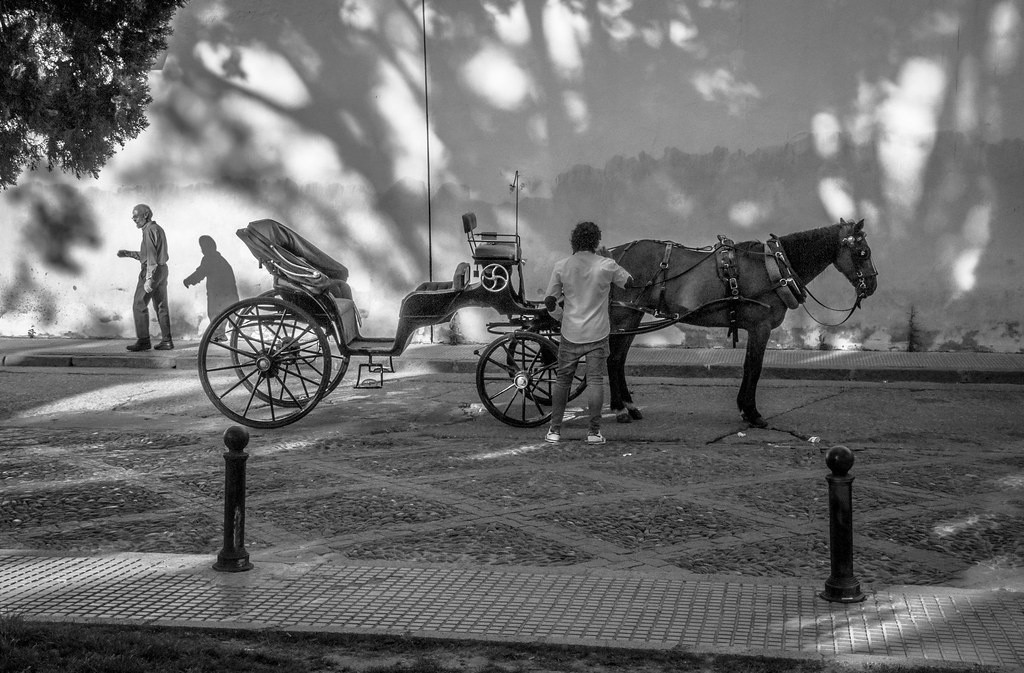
[197,212,879,430]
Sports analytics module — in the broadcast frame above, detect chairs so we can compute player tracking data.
[462,211,522,261]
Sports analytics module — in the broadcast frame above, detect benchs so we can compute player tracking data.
[400,262,470,317]
[297,257,354,314]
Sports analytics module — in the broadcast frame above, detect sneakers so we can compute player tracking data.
[154,340,174,350]
[587,430,606,444]
[545,427,560,442]
[127,337,151,350]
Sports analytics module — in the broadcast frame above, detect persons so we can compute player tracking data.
[116,202,174,351]
[545,221,634,443]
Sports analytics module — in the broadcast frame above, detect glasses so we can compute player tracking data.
[131,214,141,220]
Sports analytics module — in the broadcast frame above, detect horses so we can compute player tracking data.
[603,218,878,428]
